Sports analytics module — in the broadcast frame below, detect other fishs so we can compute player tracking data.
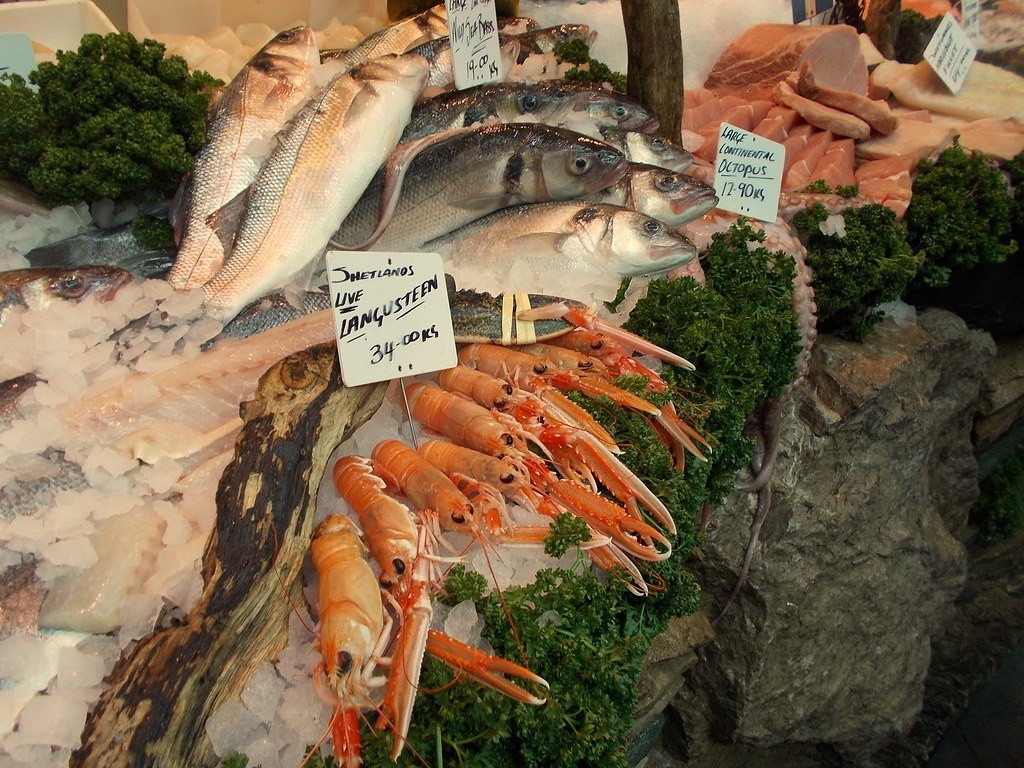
[0,0,724,641]
[68,334,393,768]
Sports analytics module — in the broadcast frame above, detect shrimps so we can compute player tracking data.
[305,297,720,768]
